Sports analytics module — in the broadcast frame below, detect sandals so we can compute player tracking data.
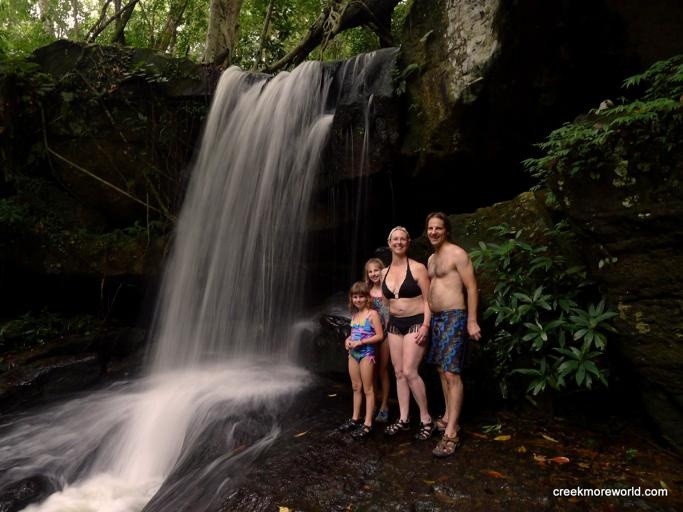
[433,417,464,435]
[351,424,375,440]
[417,417,435,444]
[338,417,363,432]
[433,433,460,457]
[386,419,412,439]
[375,408,390,426]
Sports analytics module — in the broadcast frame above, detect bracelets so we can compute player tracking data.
[361,340,365,345]
[423,324,429,328]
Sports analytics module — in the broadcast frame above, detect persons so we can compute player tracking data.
[381,226,438,441]
[425,212,483,458]
[338,281,384,440]
[363,257,389,423]
